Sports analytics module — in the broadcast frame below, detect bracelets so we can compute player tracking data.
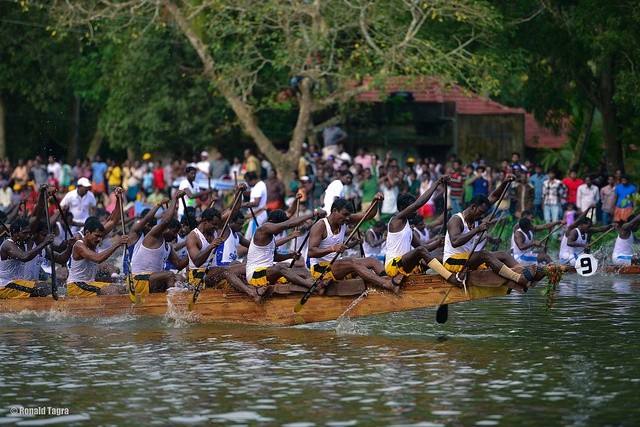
[476,226,480,233]
[331,245,335,252]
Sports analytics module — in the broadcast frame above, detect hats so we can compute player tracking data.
[77,177,92,188]
[201,151,208,157]
[407,157,415,163]
[143,153,151,160]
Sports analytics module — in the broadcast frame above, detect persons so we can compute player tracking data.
[127,190,189,304]
[59,177,96,234]
[49,205,78,247]
[246,209,333,295]
[24,183,77,282]
[323,170,353,217]
[66,193,123,281]
[0,155,65,207]
[612,214,640,267]
[234,171,268,242]
[284,125,384,187]
[270,193,303,245]
[177,166,213,224]
[185,183,271,303]
[559,216,618,268]
[210,183,251,265]
[179,149,272,180]
[263,169,286,215]
[384,151,640,211]
[49,196,98,247]
[558,203,597,257]
[164,195,222,273]
[474,207,510,251]
[361,221,387,263]
[307,192,404,294]
[123,196,171,283]
[412,205,453,245]
[0,184,57,299]
[290,200,311,259]
[511,210,567,257]
[443,173,546,292]
[512,218,553,266]
[65,186,130,295]
[64,153,179,211]
[384,175,470,288]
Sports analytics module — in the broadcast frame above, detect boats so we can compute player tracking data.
[545,265,640,274]
[0,264,566,328]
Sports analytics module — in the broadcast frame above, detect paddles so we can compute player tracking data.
[294,199,378,313]
[436,181,510,324]
[352,199,365,259]
[40,185,58,301]
[117,189,137,302]
[491,221,506,252]
[189,185,245,312]
